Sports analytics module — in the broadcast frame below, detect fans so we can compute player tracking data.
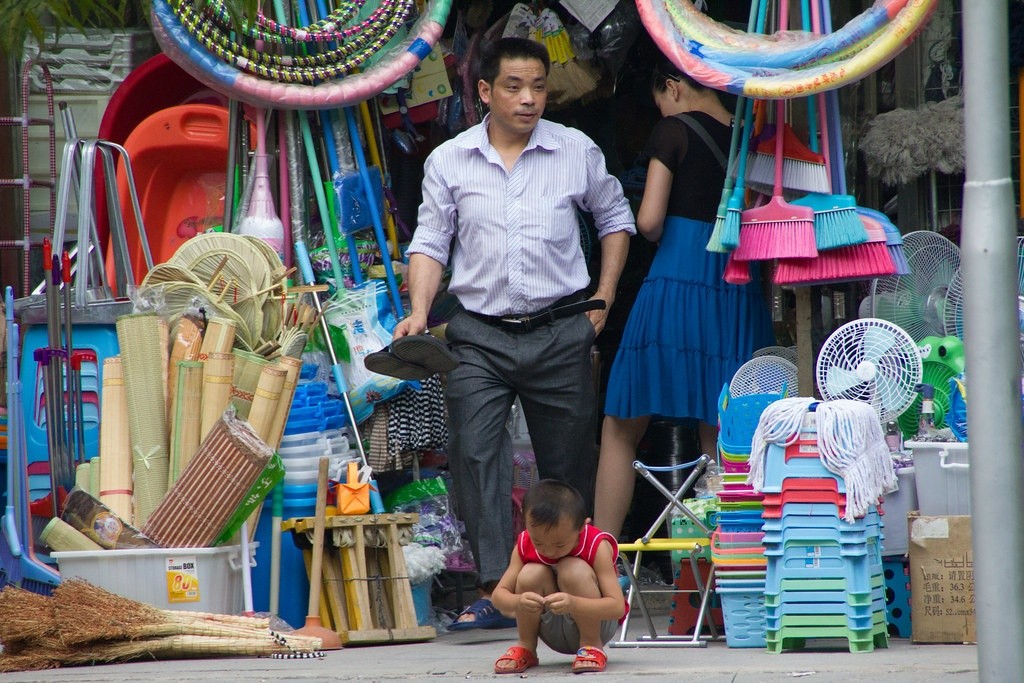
[726,228,1024,441]
[578,209,592,270]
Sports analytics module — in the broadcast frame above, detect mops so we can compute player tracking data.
[294,110,426,474]
[343,106,451,453]
[238,104,285,263]
[297,109,418,427]
[293,241,449,587]
[864,93,966,240]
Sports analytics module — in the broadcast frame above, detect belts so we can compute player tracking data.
[462,287,607,334]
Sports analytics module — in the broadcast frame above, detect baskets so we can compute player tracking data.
[714,379,790,648]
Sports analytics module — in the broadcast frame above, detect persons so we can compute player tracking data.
[491,479,630,674]
[391,39,637,630]
[594,56,777,541]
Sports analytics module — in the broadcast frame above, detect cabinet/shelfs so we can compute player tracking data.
[0,59,57,304]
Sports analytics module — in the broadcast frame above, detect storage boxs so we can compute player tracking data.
[250,362,364,629]
[16,20,160,250]
[881,466,918,556]
[710,381,799,648]
[49,541,262,618]
[907,507,978,644]
[904,428,970,517]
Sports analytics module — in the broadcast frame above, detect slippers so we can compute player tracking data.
[495,646,539,673]
[572,646,608,674]
[364,345,435,381]
[391,335,460,371]
[447,598,517,631]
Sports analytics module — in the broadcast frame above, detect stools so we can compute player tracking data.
[883,554,913,639]
[761,399,891,653]
[606,453,727,647]
[19,324,121,504]
[279,513,436,647]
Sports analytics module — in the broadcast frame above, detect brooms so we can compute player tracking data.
[505,0,602,104]
[0,235,88,599]
[0,576,322,674]
[703,0,915,285]
